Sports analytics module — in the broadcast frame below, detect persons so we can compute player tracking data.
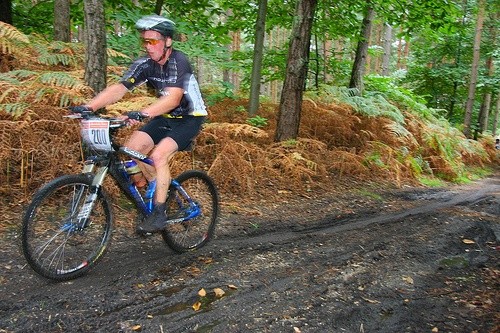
[71,16,207,231]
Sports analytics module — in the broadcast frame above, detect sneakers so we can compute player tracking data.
[137,184,150,203]
[136,205,168,234]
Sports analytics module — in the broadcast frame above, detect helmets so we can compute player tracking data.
[132,16,176,36]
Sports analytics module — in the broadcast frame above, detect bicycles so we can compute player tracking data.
[21,109,219,282]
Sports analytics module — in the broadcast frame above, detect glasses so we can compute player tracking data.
[143,38,166,45]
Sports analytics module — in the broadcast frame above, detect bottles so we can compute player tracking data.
[124,156,150,202]
